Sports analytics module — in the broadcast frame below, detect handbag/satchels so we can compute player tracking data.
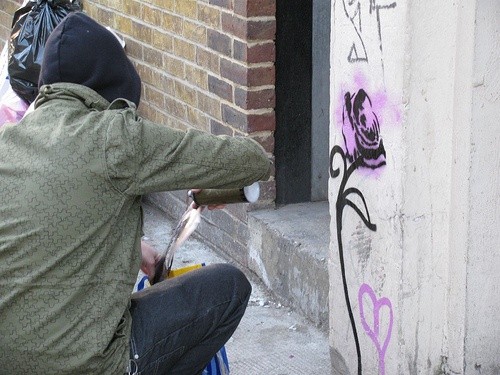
[137,263,230,375]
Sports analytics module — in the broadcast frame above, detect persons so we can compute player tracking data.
[0,13,271,375]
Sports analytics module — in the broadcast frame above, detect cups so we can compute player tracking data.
[187,182,262,209]
